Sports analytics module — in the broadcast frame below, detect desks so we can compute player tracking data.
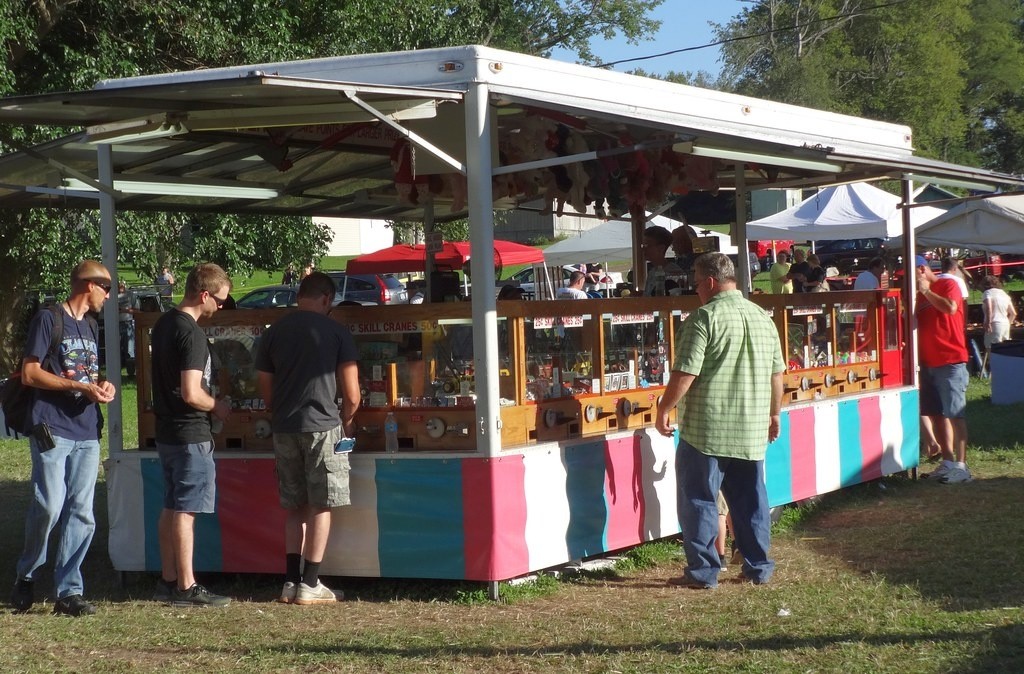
[991,339,1024,404]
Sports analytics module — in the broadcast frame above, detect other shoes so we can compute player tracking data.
[916,444,942,462]
[718,560,727,571]
[10,574,34,611]
[729,542,745,565]
[667,574,707,589]
[737,571,767,583]
[54,595,97,616]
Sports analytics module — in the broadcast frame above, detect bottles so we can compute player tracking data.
[384,412,399,454]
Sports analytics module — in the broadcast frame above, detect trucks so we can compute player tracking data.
[25,284,172,375]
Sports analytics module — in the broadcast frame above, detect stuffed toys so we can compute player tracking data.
[391,113,781,222]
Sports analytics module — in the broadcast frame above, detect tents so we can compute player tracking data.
[1,45,921,601]
[342,239,555,300]
[532,211,731,300]
[746,181,948,293]
[882,196,1024,288]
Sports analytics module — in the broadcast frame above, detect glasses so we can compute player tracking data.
[80,277,113,295]
[201,291,227,309]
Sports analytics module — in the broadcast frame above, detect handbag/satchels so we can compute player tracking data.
[811,281,830,292]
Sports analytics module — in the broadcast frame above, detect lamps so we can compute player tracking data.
[48,178,282,199]
[665,142,853,172]
[889,172,999,193]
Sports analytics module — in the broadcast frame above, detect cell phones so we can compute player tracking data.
[336,439,356,452]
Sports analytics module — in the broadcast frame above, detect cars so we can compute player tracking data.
[749,239,795,272]
[626,252,761,282]
[505,264,624,300]
[807,237,903,270]
[235,283,378,309]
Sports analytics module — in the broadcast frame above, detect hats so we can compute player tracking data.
[499,286,526,301]
[914,255,929,267]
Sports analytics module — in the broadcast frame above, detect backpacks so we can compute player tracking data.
[2,306,99,439]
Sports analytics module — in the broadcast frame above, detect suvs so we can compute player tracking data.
[317,270,409,306]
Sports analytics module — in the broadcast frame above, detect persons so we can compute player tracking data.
[217,293,237,310]
[254,273,361,605]
[118,282,142,378]
[151,264,231,609]
[624,226,698,297]
[584,263,603,292]
[156,267,174,310]
[655,252,786,588]
[894,254,973,483]
[937,257,969,334]
[281,259,314,285]
[854,257,884,321]
[557,271,589,299]
[980,275,1017,381]
[771,249,826,294]
[12,260,115,616]
[498,285,526,300]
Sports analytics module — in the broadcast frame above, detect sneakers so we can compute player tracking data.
[294,578,344,606]
[171,584,232,609]
[152,577,178,602]
[918,465,951,480]
[939,469,972,485]
[280,576,303,605]
